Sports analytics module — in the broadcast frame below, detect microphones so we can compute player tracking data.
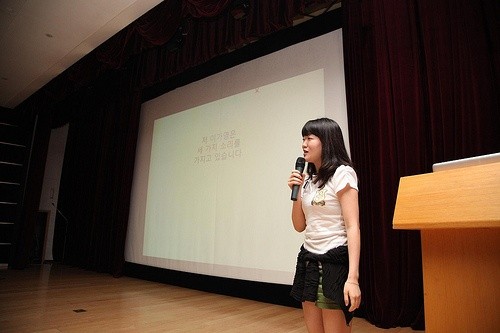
[291,157,305,201]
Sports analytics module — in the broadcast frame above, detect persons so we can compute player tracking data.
[290,118,362,333]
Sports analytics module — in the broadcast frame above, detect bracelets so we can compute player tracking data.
[347,282,359,286]
